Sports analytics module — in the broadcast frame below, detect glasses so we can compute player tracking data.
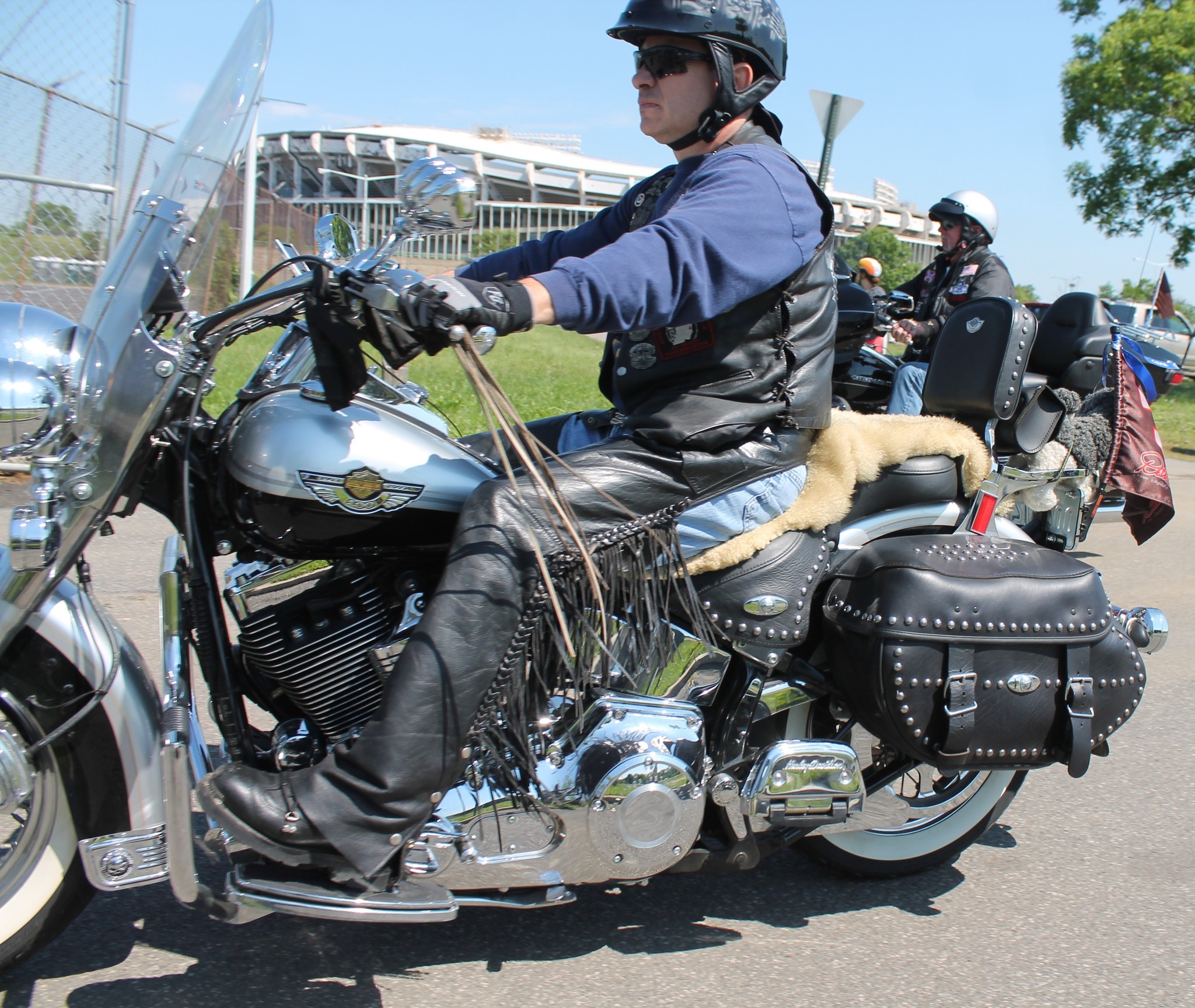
[940,219,963,231]
[633,45,747,79]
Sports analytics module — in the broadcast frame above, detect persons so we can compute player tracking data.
[884,191,1021,427]
[198,0,839,897]
[854,254,892,358]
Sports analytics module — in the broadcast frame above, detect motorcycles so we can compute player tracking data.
[0,0,1186,986]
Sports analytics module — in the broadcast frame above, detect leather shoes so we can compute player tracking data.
[199,763,365,878]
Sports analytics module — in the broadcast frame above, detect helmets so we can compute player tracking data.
[928,189,999,244]
[857,258,882,277]
[606,0,788,80]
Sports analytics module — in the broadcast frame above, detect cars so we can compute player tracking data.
[1099,297,1194,379]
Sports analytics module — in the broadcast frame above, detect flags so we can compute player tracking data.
[1099,348,1176,548]
[1154,273,1176,318]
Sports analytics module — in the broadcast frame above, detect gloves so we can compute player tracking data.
[400,275,533,357]
